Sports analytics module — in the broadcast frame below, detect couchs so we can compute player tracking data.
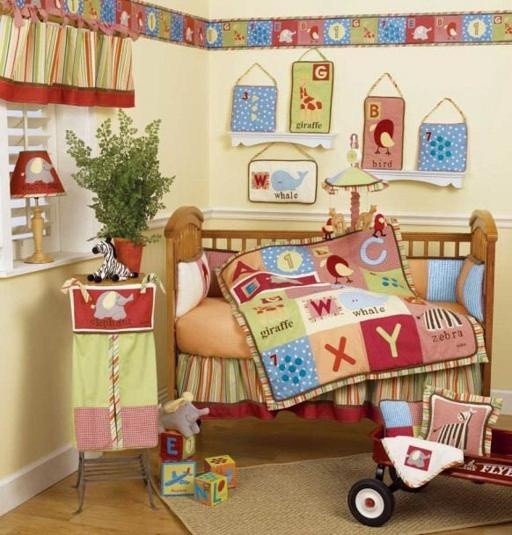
[163,205,497,432]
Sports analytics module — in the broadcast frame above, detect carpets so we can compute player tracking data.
[150,451,511,534]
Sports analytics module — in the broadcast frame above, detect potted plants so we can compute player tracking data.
[65,106,176,277]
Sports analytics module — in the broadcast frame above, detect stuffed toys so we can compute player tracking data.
[86,236,139,283]
[160,391,211,441]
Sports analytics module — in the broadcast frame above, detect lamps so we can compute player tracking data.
[10,149,67,265]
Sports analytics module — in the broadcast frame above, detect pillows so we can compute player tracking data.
[375,385,503,489]
